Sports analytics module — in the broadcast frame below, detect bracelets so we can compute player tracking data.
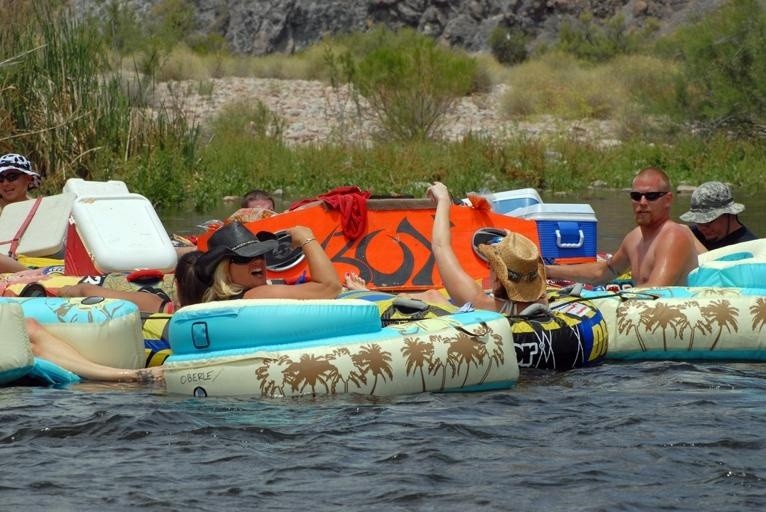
[301,237,316,248]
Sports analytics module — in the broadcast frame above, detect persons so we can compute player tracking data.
[23,318,165,384]
[1,251,26,273]
[545,167,700,289]
[344,181,549,315]
[193,222,341,304]
[53,248,210,315]
[0,153,41,219]
[240,188,277,212]
[679,180,760,256]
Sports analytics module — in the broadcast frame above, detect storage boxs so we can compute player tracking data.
[503,203,598,262]
[459,188,543,214]
[0,177,178,277]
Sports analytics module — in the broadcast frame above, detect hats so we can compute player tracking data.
[677,178,747,224]
[476,229,548,303]
[191,219,280,288]
[0,150,41,181]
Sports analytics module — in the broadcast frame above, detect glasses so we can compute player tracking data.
[0,172,26,183]
[628,189,669,203]
